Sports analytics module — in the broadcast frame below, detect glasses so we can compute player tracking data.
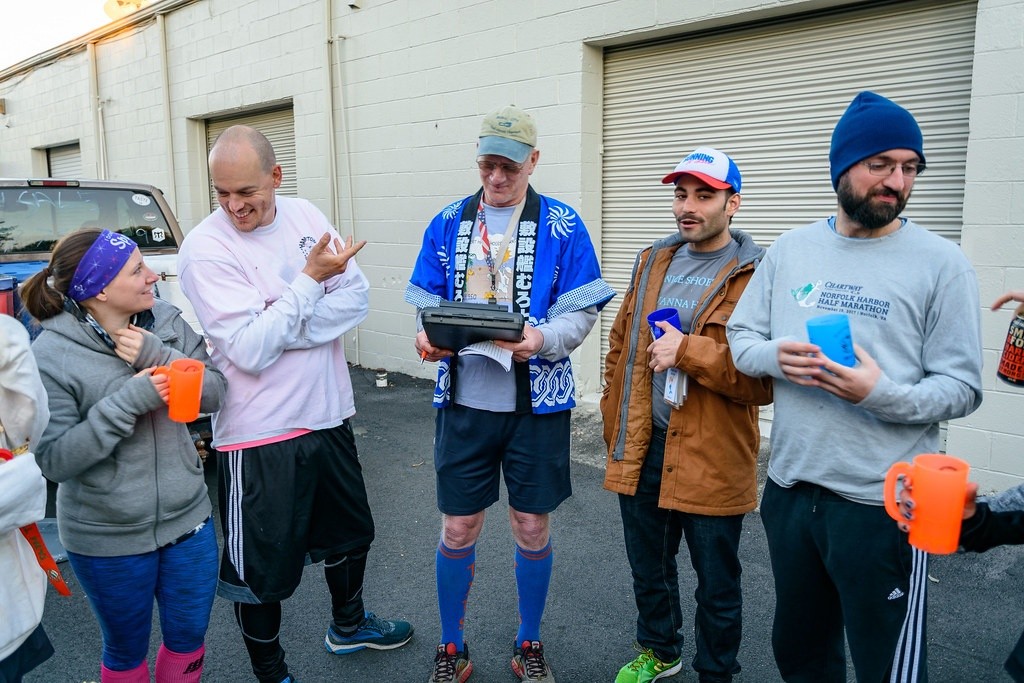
[477,159,532,178]
[861,160,927,178]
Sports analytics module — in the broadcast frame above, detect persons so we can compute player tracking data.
[177,124,415,683]
[406,107,619,683]
[724,93,984,683]
[1,310,53,683]
[896,473,1024,683]
[18,230,228,683]
[598,147,773,683]
[992,288,1024,317]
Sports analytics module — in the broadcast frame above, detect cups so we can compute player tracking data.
[884,454,970,554]
[153,358,205,423]
[806,313,856,374]
[647,308,682,341]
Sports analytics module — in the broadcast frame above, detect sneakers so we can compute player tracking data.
[614,643,682,683]
[511,633,556,683]
[428,637,471,683]
[324,609,415,655]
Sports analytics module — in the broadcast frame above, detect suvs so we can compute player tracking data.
[0,176,206,344]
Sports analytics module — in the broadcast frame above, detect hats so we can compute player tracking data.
[829,92,926,192]
[477,104,536,163]
[662,147,742,194]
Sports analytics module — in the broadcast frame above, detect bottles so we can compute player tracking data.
[376,369,388,387]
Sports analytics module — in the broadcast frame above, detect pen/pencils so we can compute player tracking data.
[421,350,428,365]
